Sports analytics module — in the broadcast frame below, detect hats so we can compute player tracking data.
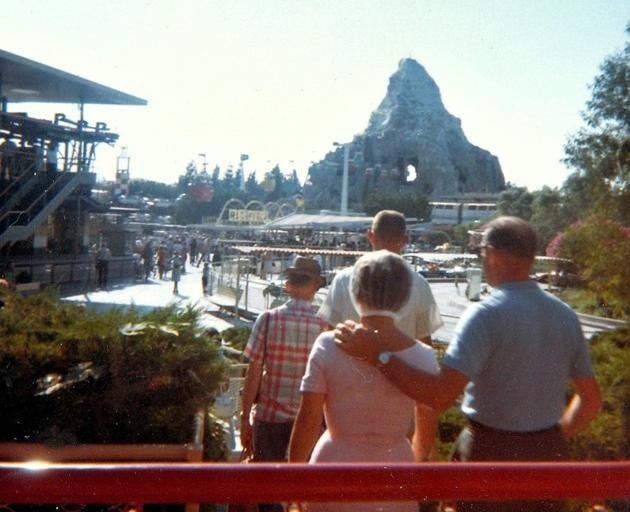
[283,256,327,288]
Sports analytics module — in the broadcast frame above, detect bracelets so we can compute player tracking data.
[241,414,250,422]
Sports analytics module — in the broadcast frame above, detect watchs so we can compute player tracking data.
[375,351,394,372]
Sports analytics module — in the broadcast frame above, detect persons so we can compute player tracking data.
[123,226,421,295]
[95,243,112,287]
[0,135,18,183]
[45,139,59,187]
[0,229,80,308]
[195,326,238,452]
[314,207,445,350]
[84,241,99,284]
[238,254,335,511]
[327,215,603,512]
[282,248,445,511]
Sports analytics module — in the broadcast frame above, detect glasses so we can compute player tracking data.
[472,245,491,254]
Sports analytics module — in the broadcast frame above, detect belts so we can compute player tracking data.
[467,419,558,436]
